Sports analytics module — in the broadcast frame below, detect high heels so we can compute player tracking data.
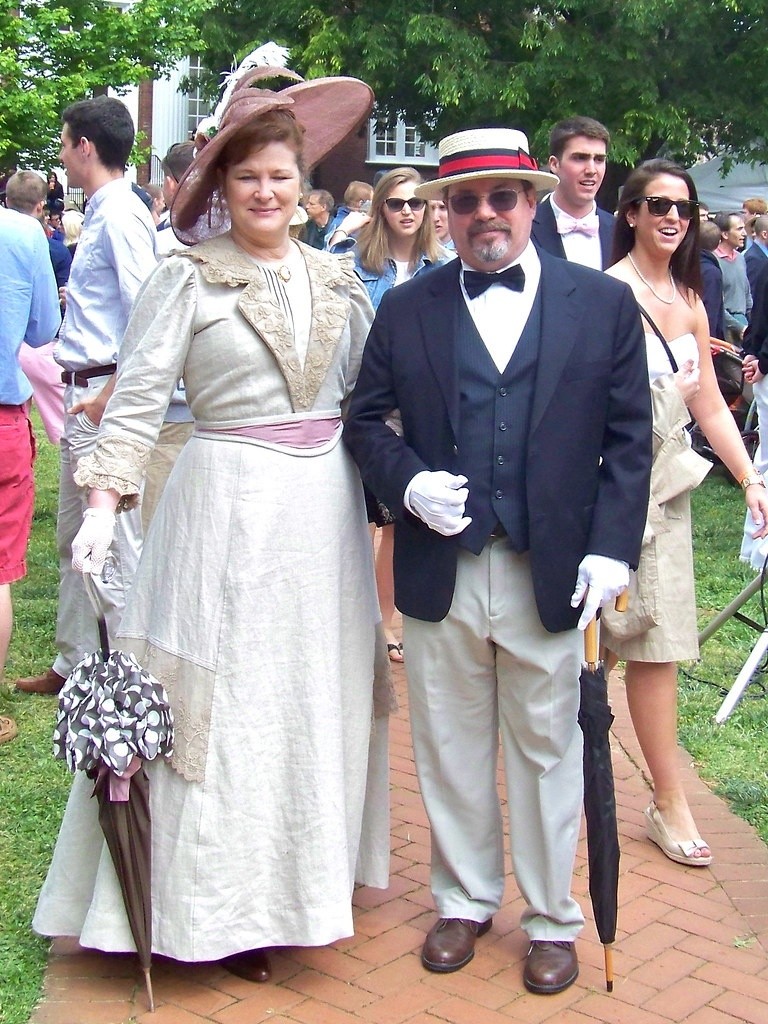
[643,799,713,866]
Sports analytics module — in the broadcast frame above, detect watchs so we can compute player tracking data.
[741,474,767,492]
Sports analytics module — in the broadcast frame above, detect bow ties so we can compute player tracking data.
[463,263,525,300]
[556,213,600,238]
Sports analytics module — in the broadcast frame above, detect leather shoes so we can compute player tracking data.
[14,667,67,695]
[523,939,580,994]
[216,947,272,983]
[421,913,493,972]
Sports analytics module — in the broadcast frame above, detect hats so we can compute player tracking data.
[168,42,376,249]
[289,206,309,226]
[413,127,561,202]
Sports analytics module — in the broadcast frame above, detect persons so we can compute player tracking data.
[15,98,167,695]
[34,44,404,982]
[530,116,618,272]
[290,168,456,664]
[0,206,63,745]
[344,129,654,994]
[140,141,198,535]
[4,171,85,446]
[685,197,768,570]
[602,160,768,865]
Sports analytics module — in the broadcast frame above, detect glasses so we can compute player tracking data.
[636,196,701,221]
[381,197,427,212]
[444,188,527,215]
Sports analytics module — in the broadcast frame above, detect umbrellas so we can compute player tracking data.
[54,552,174,1013]
[577,587,628,993]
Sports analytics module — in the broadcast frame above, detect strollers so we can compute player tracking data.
[682,346,761,489]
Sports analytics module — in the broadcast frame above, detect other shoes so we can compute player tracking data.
[0,715,18,745]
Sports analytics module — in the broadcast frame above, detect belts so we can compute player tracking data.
[60,363,117,387]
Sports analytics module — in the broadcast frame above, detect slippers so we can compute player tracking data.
[384,640,407,663]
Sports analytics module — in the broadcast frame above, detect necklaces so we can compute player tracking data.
[628,253,676,304]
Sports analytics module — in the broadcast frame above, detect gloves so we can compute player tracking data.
[408,470,473,537]
[70,508,115,576]
[379,408,405,437]
[569,552,630,631]
